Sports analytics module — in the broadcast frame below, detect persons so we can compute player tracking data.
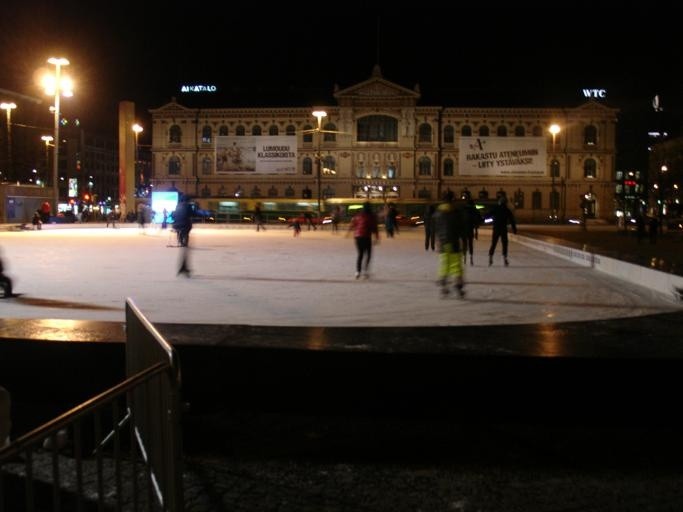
[345,201,379,279]
[138,204,145,230]
[254,202,266,231]
[420,191,517,295]
[287,206,341,238]
[649,218,657,244]
[36,197,116,229]
[636,218,645,244]
[163,192,205,275]
[384,203,398,239]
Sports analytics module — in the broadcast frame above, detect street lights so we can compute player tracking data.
[39,57,72,204]
[1,101,17,180]
[130,124,144,187]
[313,109,328,211]
[549,125,560,221]
